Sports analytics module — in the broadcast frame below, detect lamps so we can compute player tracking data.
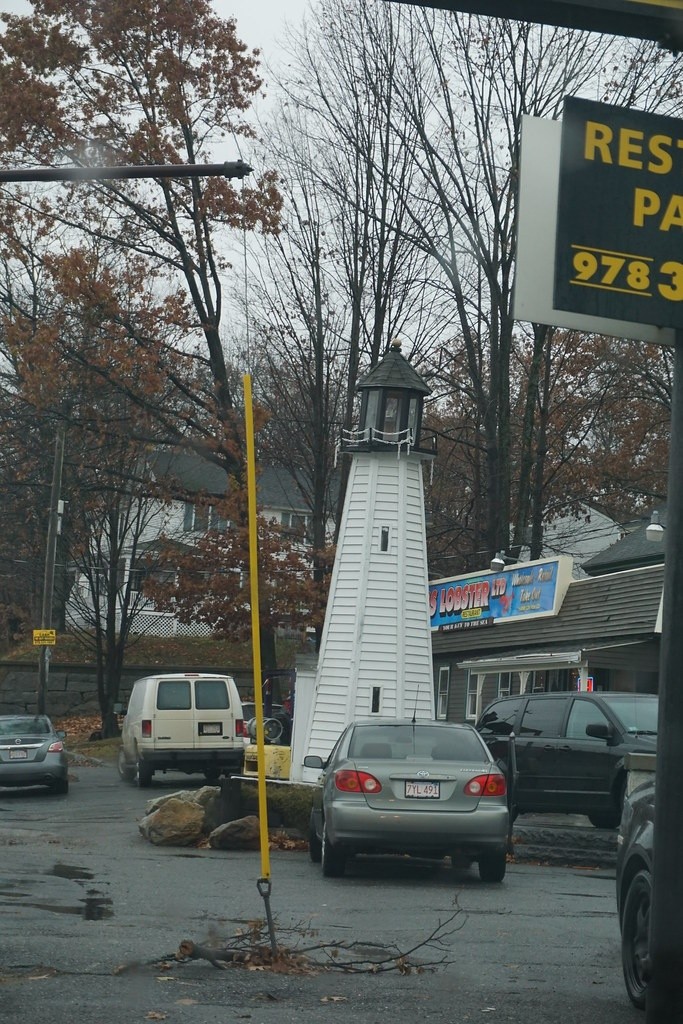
[490,552,524,572]
[645,510,666,542]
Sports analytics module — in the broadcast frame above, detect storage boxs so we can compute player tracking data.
[243,744,291,781]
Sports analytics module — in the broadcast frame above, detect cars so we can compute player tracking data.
[300,686,511,885]
[0,714,69,794]
[239,700,288,760]
[613,777,656,1007]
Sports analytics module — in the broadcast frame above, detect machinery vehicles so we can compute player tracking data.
[235,667,295,784]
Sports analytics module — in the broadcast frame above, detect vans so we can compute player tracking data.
[119,673,244,781]
[472,690,658,832]
